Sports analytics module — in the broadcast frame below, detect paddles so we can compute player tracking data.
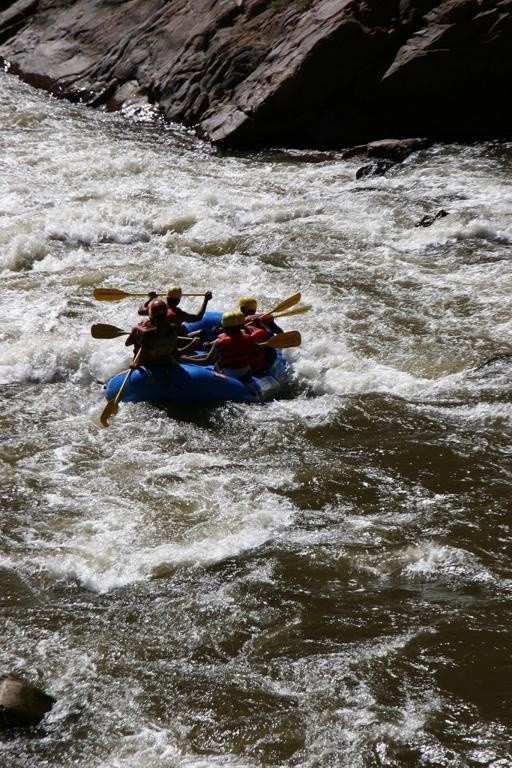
[204,292,312,347]
[92,323,194,341]
[100,345,144,428]
[93,288,209,301]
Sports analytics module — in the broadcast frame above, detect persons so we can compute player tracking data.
[125,299,201,365]
[137,286,213,341]
[211,296,274,339]
[203,313,284,377]
[179,311,268,383]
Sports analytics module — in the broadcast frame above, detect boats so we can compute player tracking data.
[105,310,299,418]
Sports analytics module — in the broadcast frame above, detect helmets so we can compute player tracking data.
[166,286,182,299]
[239,297,257,310]
[149,301,167,320]
[222,310,245,327]
